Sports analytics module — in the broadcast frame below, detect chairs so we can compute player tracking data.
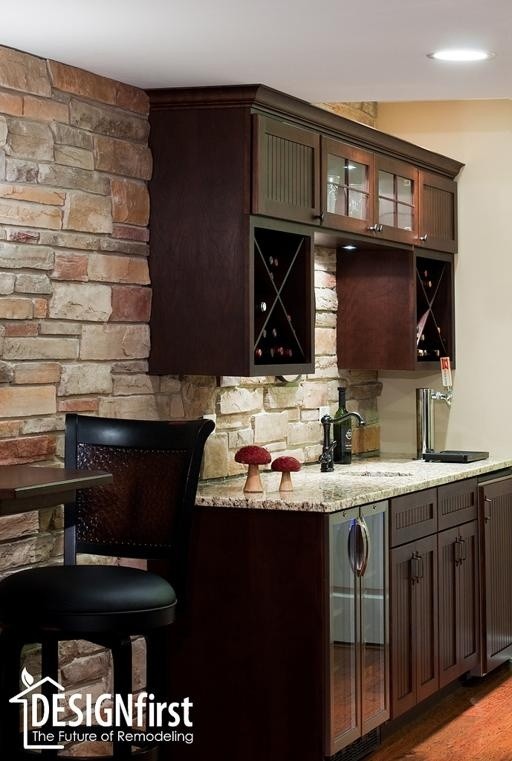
[0,412,217,761]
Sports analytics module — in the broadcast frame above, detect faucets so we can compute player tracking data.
[321,412,365,471]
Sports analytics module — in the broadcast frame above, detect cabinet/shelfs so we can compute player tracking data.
[470,474,511,678]
[321,132,419,254]
[146,502,392,760]
[146,111,324,379]
[338,172,458,369]
[391,477,481,721]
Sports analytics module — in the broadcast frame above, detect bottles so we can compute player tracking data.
[256,253,295,360]
[416,270,442,359]
[333,387,354,461]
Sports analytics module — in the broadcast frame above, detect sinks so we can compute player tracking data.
[342,473,413,477]
